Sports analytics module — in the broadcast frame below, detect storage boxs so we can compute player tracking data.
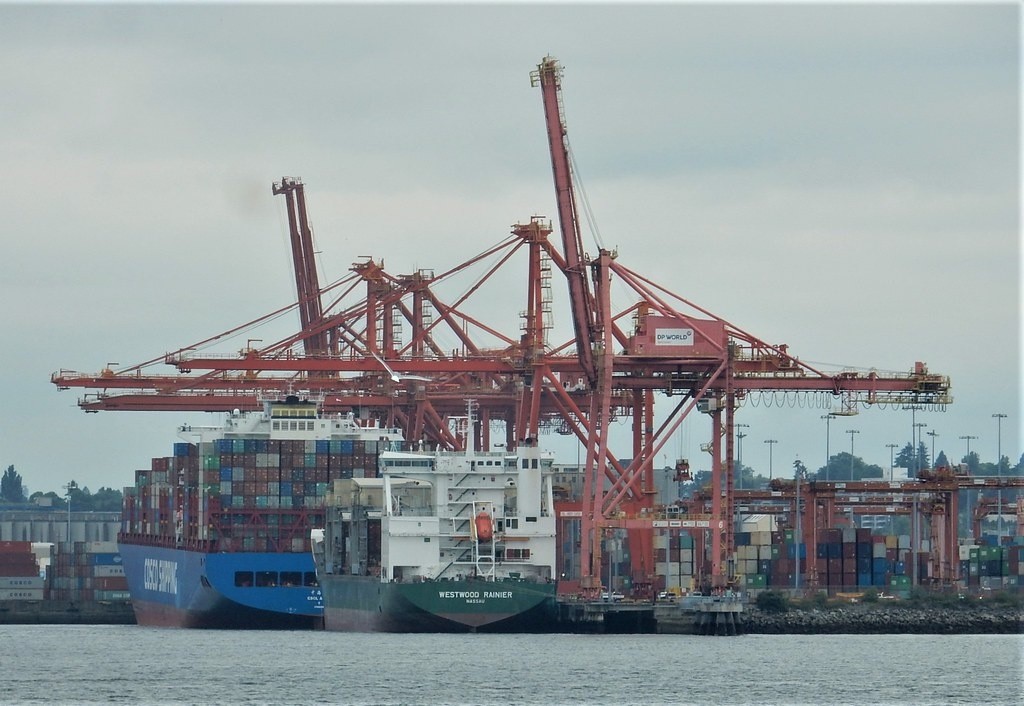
[566,528,1024,597]
[46,439,437,600]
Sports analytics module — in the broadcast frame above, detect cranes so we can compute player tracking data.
[49,54,955,590]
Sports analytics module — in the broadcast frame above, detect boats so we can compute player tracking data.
[308,396,565,629]
[114,388,402,631]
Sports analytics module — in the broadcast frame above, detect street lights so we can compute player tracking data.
[845,428,860,480]
[820,414,836,482]
[959,435,977,535]
[735,423,749,493]
[763,439,778,481]
[886,442,900,483]
[991,413,1008,546]
[914,422,927,474]
[928,430,939,473]
[901,406,921,480]
